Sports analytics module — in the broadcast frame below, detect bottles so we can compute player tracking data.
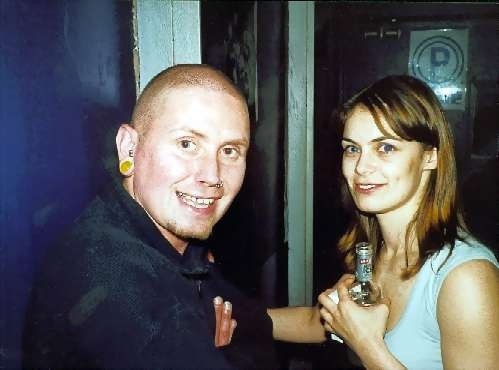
[348,242,382,306]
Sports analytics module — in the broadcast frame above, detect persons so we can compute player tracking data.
[25,65,275,370]
[266,74,499,370]
